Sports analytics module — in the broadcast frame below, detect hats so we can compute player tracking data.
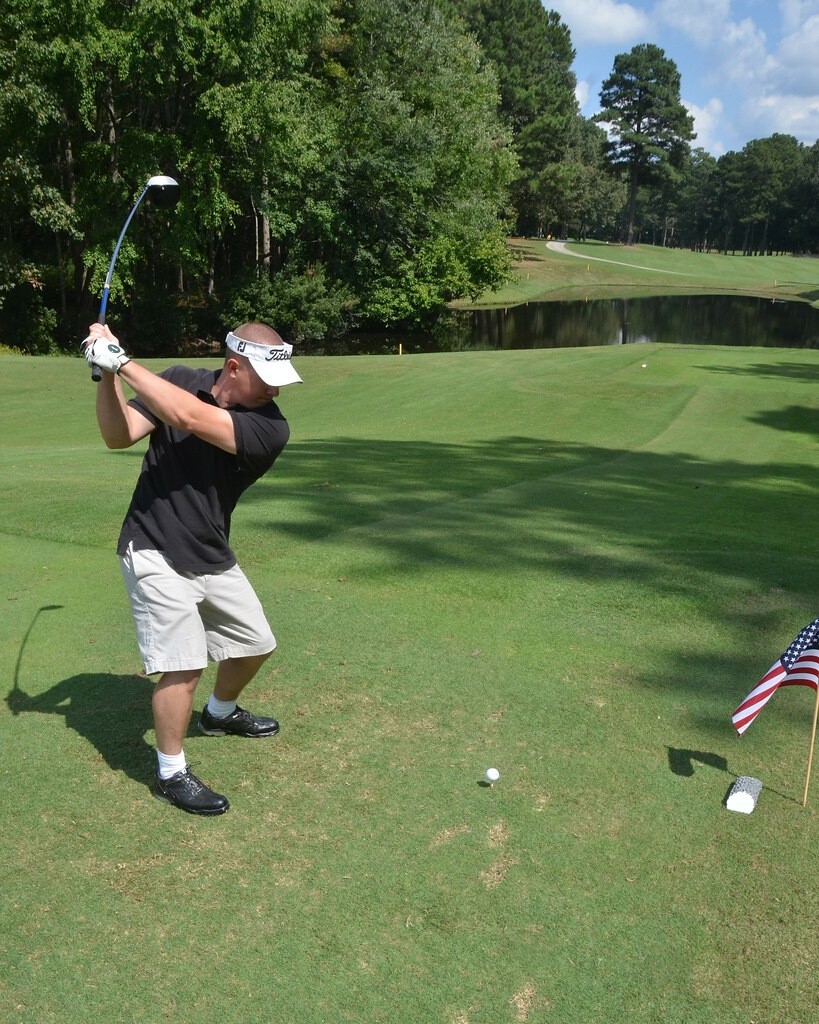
[225,332,305,387]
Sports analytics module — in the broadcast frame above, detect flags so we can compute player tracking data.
[731,617,819,737]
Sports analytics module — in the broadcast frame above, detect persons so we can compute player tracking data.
[82,321,304,815]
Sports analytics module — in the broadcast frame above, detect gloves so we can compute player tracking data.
[84,337,131,376]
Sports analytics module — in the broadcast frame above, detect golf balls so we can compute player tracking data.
[486,768,499,781]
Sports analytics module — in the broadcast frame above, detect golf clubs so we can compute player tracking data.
[91,175,181,383]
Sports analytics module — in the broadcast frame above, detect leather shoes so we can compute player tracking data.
[195,704,280,737]
[150,761,232,817]
